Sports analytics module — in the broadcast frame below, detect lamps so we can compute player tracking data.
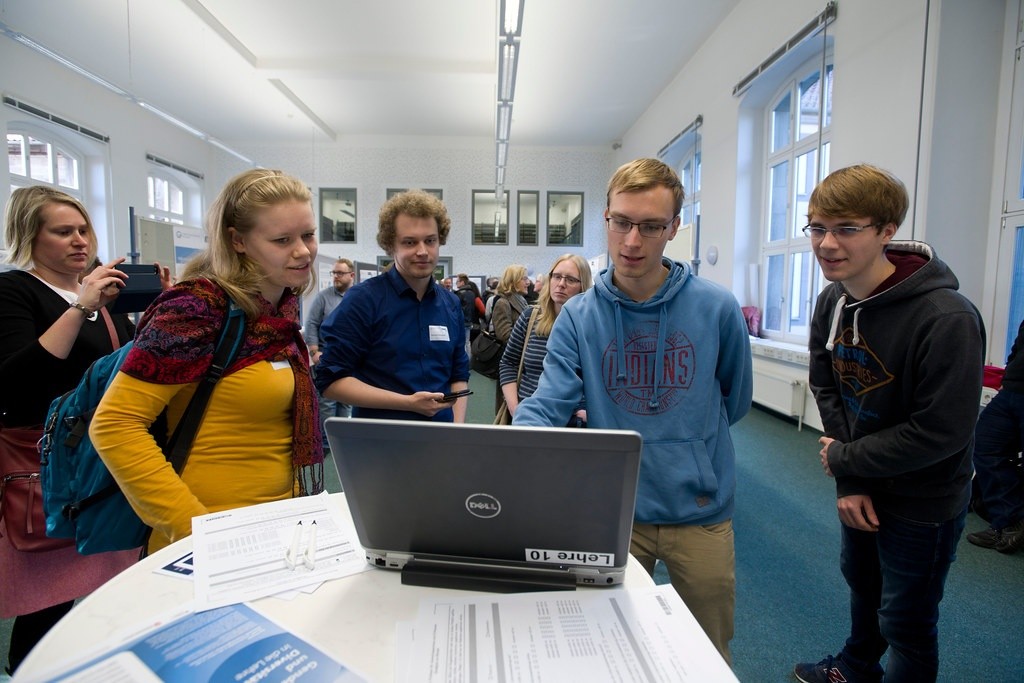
[495,0,526,198]
[706,246,718,266]
[0,0,254,163]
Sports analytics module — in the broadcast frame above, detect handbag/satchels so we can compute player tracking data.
[0,430,74,552]
[473,294,513,381]
[489,398,515,427]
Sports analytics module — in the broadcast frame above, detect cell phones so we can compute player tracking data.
[115,262,163,291]
[438,389,472,403]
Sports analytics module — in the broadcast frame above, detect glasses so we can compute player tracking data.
[605,213,677,238]
[550,273,581,286]
[803,223,875,238]
[329,270,349,278]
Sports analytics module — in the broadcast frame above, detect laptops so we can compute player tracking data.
[325,417,642,585]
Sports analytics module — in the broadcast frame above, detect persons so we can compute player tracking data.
[512,158,752,667]
[492,264,530,415]
[0,187,172,671]
[967,318,1024,554]
[314,189,472,422]
[445,274,547,345]
[499,254,593,427]
[89,169,325,556]
[795,164,987,683]
[306,258,355,456]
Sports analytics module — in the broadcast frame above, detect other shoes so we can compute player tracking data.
[966,524,1001,550]
[995,528,1024,553]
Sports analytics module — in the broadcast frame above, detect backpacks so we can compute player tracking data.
[40,299,245,554]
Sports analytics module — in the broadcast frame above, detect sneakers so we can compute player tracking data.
[794,654,865,683]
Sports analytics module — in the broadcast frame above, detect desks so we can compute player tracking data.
[9,492,741,683]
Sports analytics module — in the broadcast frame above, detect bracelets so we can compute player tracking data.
[69,300,95,318]
